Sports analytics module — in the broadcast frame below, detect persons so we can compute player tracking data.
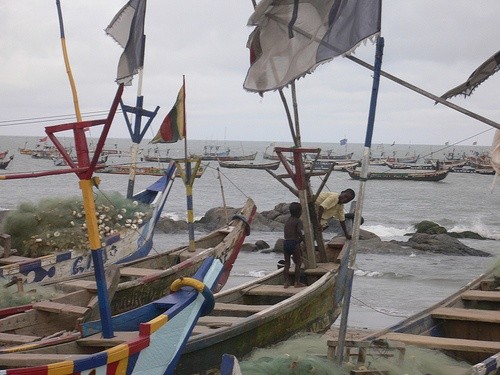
[299,189,355,266]
[283,202,307,287]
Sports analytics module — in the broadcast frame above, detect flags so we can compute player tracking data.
[340,139,346,145]
[150,85,186,144]
[242,1,382,92]
[434,51,500,104]
[40,137,47,142]
[106,0,147,85]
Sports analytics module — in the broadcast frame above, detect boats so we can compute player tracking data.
[0,127,500,176]
[1,76,242,356]
[0,32,178,294]
[175,5,359,375]
[242,4,500,375]
[0,0,255,375]
[346,157,449,181]
